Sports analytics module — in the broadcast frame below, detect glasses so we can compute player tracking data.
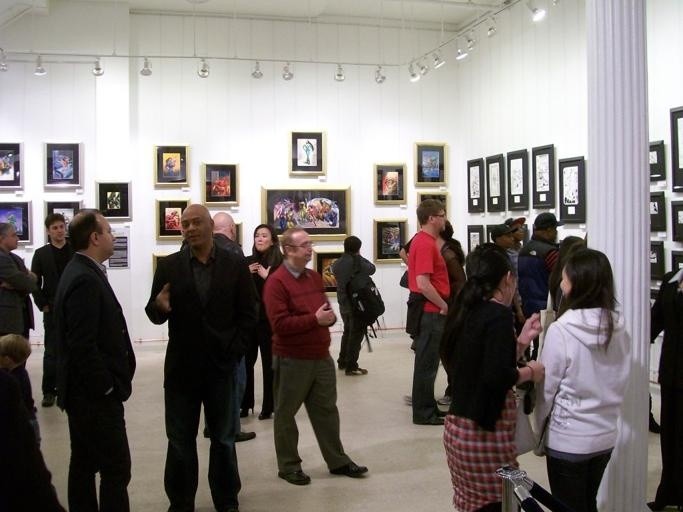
[290,242,313,249]
[435,214,446,218]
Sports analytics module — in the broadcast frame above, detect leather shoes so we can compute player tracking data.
[330,461,368,475]
[42,391,56,407]
[234,432,256,442]
[278,471,311,486]
[437,408,448,417]
[650,415,661,433]
[412,417,445,425]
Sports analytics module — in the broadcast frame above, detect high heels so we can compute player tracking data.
[239,408,254,417]
[258,410,274,420]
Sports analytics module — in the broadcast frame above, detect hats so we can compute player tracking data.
[491,224,518,240]
[504,216,526,226]
[532,213,565,231]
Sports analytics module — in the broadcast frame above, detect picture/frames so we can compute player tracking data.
[0,140,25,192]
[153,253,169,277]
[0,201,31,248]
[43,201,80,235]
[233,221,243,253]
[93,180,134,222]
[200,161,241,207]
[648,104,682,280]
[256,129,453,299]
[464,143,587,254]
[44,142,81,188]
[154,198,191,240]
[152,143,190,188]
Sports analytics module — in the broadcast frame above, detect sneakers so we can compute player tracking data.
[338,362,359,370]
[437,396,452,405]
[345,368,367,375]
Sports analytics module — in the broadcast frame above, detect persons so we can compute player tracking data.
[261,228,368,486]
[143,204,261,510]
[442,241,547,511]
[649,396,661,433]
[491,213,588,365]
[400,221,467,405]
[333,236,376,375]
[47,208,136,510]
[405,198,452,426]
[650,267,683,509]
[213,212,286,419]
[0,222,38,342]
[29,212,75,408]
[0,334,42,446]
[536,248,633,511]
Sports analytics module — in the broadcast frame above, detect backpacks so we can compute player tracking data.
[348,263,385,325]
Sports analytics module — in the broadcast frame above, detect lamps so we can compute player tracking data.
[0,0,395,87]
[402,0,555,84]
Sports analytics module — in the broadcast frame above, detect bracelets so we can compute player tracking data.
[525,364,535,379]
